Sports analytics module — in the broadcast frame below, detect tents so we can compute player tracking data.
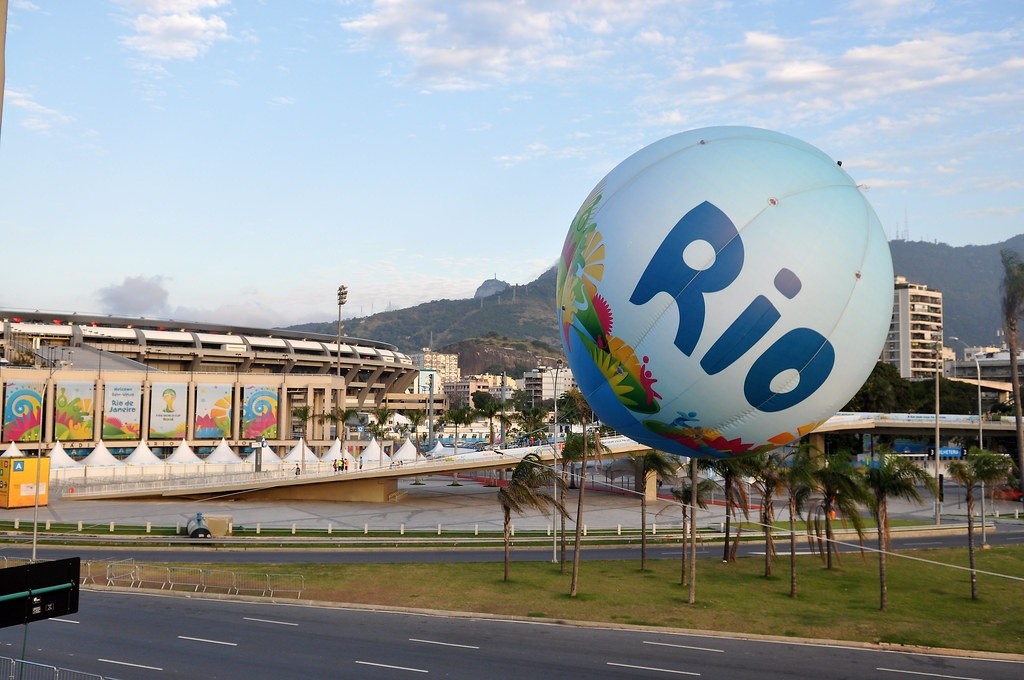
[0,439,448,467]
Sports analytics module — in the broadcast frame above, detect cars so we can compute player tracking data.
[989,484,1024,502]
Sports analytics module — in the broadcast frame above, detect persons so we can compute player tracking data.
[295,463,301,475]
[333,457,348,472]
[359,456,363,469]
[519,436,542,447]
[390,461,404,469]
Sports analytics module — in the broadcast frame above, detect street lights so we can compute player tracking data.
[32,359,73,563]
[499,370,506,450]
[551,358,564,564]
[6,325,197,383]
[948,335,986,546]
[235,353,242,383]
[336,284,349,450]
[931,332,942,527]
[428,373,434,458]
[282,353,289,383]
